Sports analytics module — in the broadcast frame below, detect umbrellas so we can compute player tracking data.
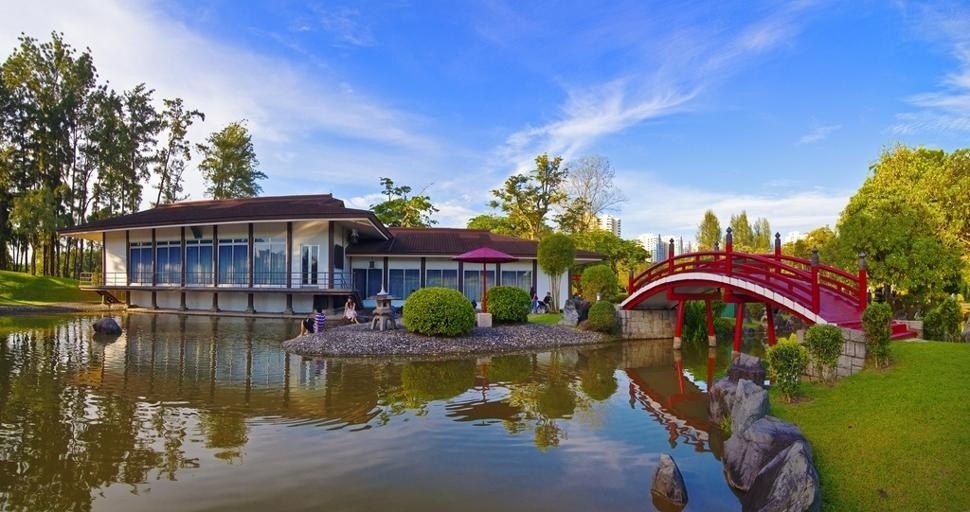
[449,246,519,313]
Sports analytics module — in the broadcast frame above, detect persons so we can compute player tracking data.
[343,296,362,325]
[300,355,325,376]
[543,290,553,312]
[471,299,478,310]
[529,286,538,314]
[298,304,328,336]
